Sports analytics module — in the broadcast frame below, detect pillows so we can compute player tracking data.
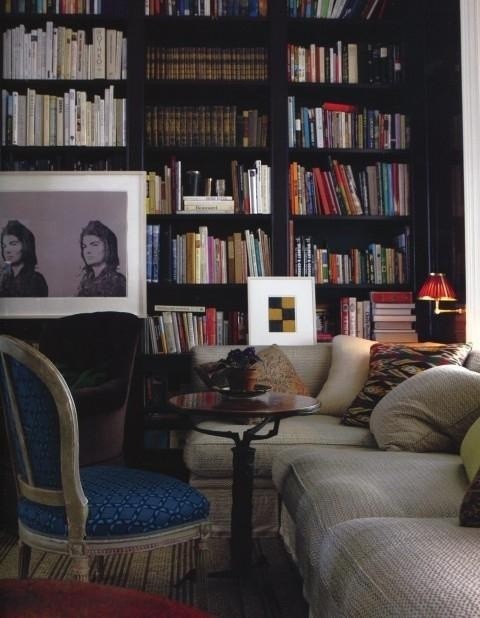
[193,343,313,424]
[315,334,378,416]
[459,467,480,527]
[368,363,480,453]
[459,415,480,484]
[340,341,472,428]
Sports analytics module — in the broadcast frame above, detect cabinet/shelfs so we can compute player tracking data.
[0,0,440,454]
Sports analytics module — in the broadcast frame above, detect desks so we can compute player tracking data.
[170,391,322,577]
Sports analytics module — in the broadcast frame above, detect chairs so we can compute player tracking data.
[0,333,213,586]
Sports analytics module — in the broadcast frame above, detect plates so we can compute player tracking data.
[212,383,273,400]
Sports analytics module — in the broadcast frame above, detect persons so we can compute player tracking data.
[320,311,331,331]
[77,221,126,297]
[0,219,48,297]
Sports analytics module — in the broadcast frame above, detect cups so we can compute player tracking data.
[229,309,246,345]
[184,169,226,196]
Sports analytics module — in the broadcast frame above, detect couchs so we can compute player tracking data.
[182,342,480,618]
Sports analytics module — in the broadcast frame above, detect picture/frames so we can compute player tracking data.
[246,275,318,348]
[0,168,148,320]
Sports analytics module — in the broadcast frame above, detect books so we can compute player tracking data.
[288,96,410,149]
[288,39,401,84]
[290,220,405,284]
[0,0,127,171]
[288,0,386,19]
[146,47,268,80]
[145,305,247,354]
[146,225,271,284]
[337,293,418,343]
[146,153,271,214]
[145,0,267,16]
[289,155,411,216]
[146,105,269,147]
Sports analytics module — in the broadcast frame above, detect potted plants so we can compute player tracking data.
[216,347,262,394]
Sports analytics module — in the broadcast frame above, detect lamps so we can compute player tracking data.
[417,273,464,315]
